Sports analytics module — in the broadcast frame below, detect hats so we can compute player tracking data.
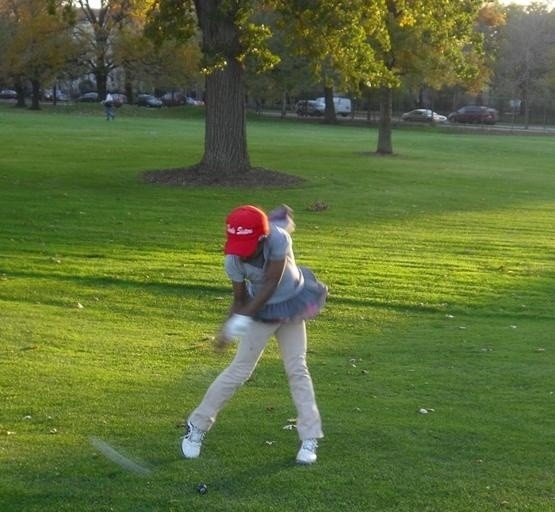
[225,205,269,257]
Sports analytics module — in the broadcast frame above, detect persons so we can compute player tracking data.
[103,91,116,121]
[180,202,330,465]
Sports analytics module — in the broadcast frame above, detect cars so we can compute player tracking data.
[448,104,500,125]
[41,90,67,101]
[400,109,447,124]
[77,88,204,108]
[0,90,18,97]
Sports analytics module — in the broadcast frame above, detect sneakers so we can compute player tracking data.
[296,439,317,463]
[180,421,204,457]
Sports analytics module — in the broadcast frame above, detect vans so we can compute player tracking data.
[297,97,351,116]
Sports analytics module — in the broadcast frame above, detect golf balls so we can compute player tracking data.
[195,485,207,495]
